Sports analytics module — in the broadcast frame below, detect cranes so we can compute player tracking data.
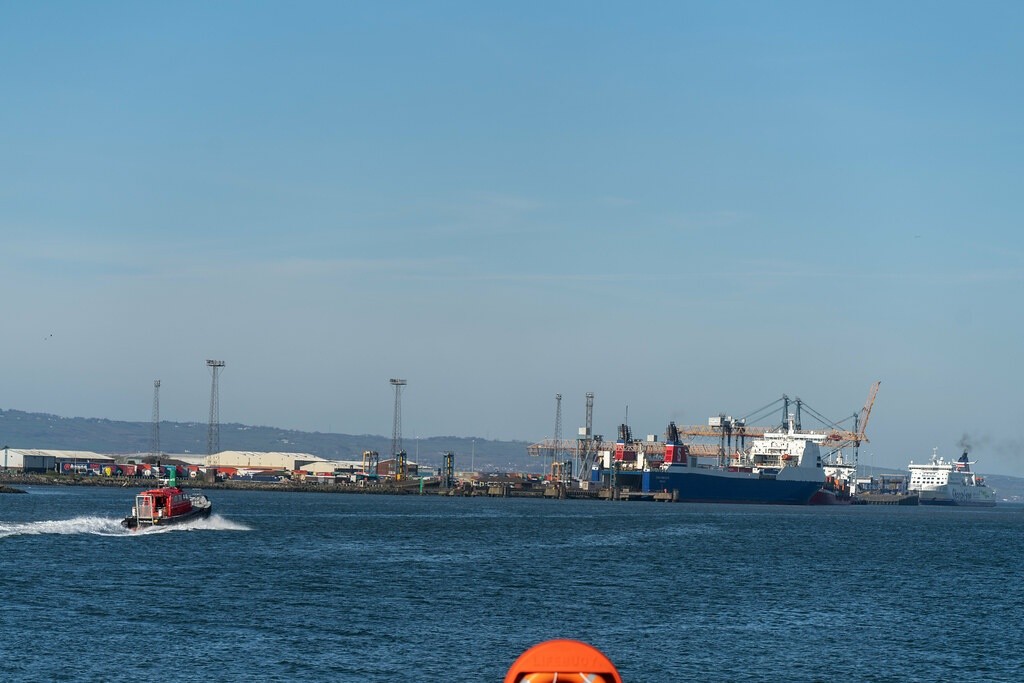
[673,378,882,468]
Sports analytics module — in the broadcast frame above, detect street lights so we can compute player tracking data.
[472,439,475,473]
[416,436,419,467]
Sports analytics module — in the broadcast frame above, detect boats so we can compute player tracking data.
[906,446,997,507]
[606,413,857,506]
[119,477,213,533]
[782,454,793,460]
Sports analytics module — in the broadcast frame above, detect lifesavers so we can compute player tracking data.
[519,672,608,683]
